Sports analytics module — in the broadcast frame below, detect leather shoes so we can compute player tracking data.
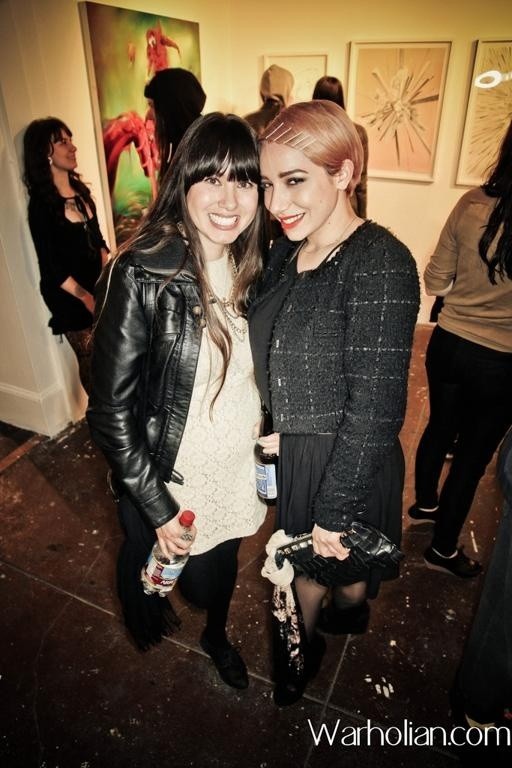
[200,631,249,690]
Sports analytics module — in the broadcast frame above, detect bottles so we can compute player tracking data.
[254,404,279,505]
[141,511,197,597]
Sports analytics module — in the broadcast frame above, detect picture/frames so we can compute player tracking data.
[455,38,512,190]
[79,3,200,257]
[260,54,328,106]
[344,39,452,184]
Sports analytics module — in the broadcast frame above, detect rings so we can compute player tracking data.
[180,526,194,542]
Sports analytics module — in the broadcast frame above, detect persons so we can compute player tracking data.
[248,101,421,704]
[312,77,367,220]
[408,117,512,578]
[86,113,269,687]
[21,118,110,397]
[145,68,207,188]
[244,65,294,135]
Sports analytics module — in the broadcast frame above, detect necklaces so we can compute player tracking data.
[300,217,357,278]
[177,220,246,345]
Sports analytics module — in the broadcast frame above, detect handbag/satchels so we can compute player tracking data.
[274,521,405,591]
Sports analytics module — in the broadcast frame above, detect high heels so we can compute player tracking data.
[273,635,328,706]
[318,594,370,635]
[423,546,481,578]
[406,504,439,526]
